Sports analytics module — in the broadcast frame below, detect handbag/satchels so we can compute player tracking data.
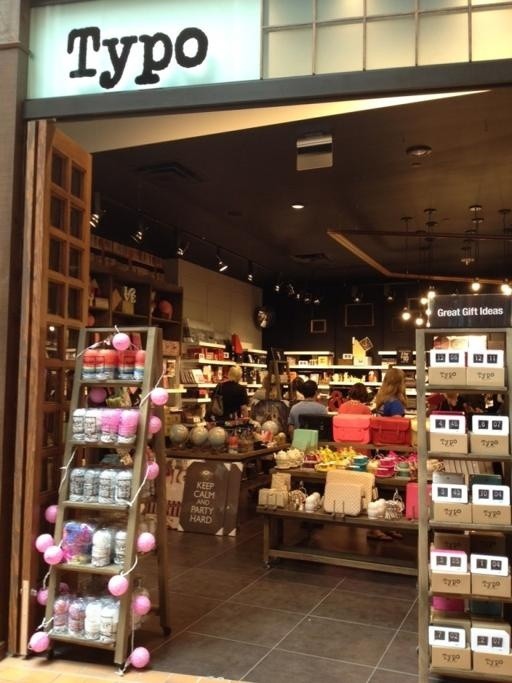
[331,415,371,445]
[411,417,445,447]
[372,417,412,444]
[291,430,319,449]
[298,413,332,442]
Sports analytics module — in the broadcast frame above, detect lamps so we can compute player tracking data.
[497,208,510,294]
[175,226,191,259]
[246,257,255,284]
[89,191,106,232]
[128,209,148,249]
[210,244,229,273]
[398,209,439,330]
[272,269,283,293]
[468,204,483,294]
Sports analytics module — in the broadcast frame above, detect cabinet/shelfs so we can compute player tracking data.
[415,327,512,682]
[45,326,172,673]
[255,441,445,578]
[174,339,269,429]
[280,352,417,396]
[163,443,292,537]
[86,267,186,451]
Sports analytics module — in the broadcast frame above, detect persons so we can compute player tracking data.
[338,383,372,415]
[288,380,328,436]
[250,373,288,435]
[211,365,249,421]
[375,368,408,417]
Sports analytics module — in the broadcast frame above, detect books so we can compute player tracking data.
[180,365,225,384]
[318,356,334,365]
[284,371,297,382]
[443,459,495,485]
[286,356,300,365]
